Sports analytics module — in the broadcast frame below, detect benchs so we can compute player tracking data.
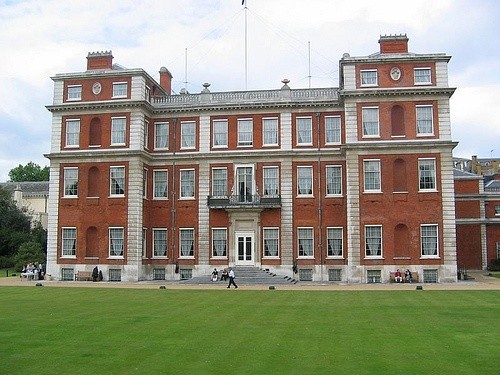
[75,270,92,281]
[390,272,417,282]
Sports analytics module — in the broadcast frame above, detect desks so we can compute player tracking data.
[21,273,38,280]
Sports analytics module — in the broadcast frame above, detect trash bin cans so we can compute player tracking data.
[458,273,464,280]
[48,275,51,281]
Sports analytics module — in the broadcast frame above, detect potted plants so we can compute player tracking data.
[47,274,52,280]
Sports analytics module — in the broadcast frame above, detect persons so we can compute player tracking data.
[394,269,403,283]
[92,266,103,282]
[404,269,413,283]
[210,268,218,281]
[227,267,238,288]
[220,269,229,281]
[22,262,45,280]
